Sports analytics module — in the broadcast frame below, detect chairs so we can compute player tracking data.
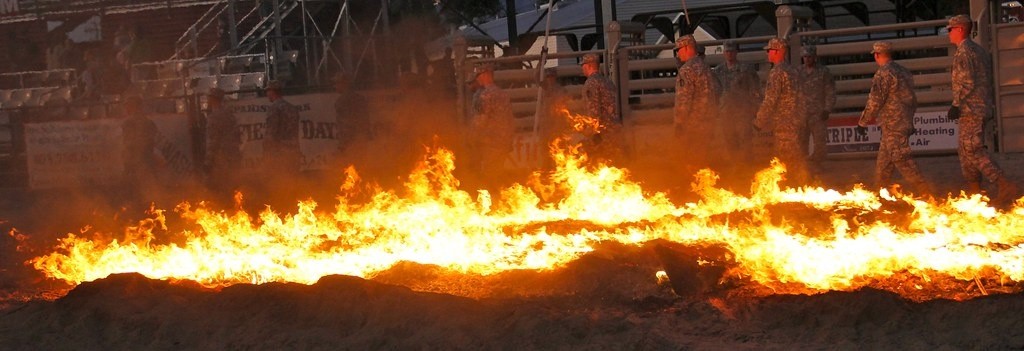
[0,50,299,152]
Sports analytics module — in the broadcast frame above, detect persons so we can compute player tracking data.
[333,72,370,155]
[858,40,932,196]
[463,63,515,138]
[672,34,836,164]
[439,46,458,99]
[579,51,618,126]
[122,95,158,200]
[202,87,241,175]
[534,47,568,174]
[262,80,301,178]
[942,14,1019,206]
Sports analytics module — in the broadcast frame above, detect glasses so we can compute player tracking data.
[947,27,959,32]
[766,49,778,53]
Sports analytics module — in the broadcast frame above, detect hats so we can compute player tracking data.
[123,94,143,105]
[543,67,557,78]
[262,79,284,90]
[944,14,973,29]
[330,72,350,83]
[763,38,789,50]
[869,42,893,54]
[673,34,697,51]
[205,88,225,101]
[799,46,817,57]
[471,64,494,78]
[578,53,601,64]
[399,71,417,82]
[723,39,738,51]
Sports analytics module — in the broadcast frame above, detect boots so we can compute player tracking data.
[987,177,1021,206]
[964,181,980,198]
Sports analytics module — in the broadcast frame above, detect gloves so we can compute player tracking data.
[855,125,869,135]
[675,123,684,138]
[593,134,602,145]
[202,166,211,175]
[947,105,962,120]
[753,125,762,132]
[822,110,829,121]
